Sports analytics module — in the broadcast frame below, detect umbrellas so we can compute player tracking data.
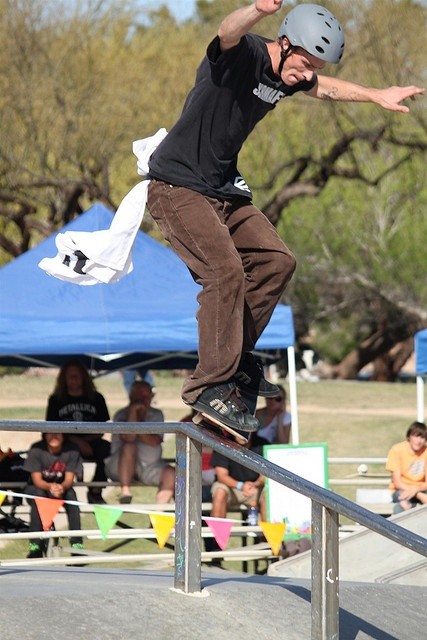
[414,328,427,440]
[2,204,299,445]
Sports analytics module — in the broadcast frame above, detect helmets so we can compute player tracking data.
[277,3,345,64]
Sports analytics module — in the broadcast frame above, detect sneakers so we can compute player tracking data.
[187,378,261,433]
[26,543,43,558]
[232,355,280,399]
[71,543,84,550]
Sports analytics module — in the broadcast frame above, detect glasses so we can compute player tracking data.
[267,396,284,402]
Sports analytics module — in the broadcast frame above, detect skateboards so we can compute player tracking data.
[191,354,263,446]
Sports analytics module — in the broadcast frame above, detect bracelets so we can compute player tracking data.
[237,481,243,491]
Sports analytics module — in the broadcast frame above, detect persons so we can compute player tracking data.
[44,363,112,506]
[386,421,427,512]
[208,427,266,529]
[104,381,176,539]
[247,383,291,452]
[145,1,425,446]
[24,431,89,569]
